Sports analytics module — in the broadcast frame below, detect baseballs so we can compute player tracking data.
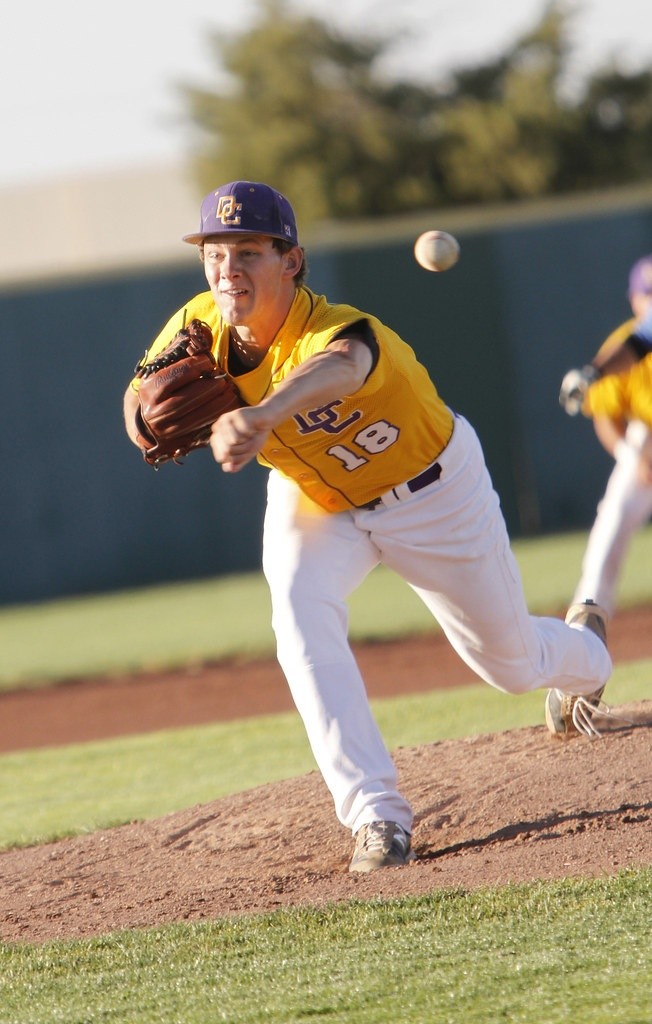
[413,231,459,272]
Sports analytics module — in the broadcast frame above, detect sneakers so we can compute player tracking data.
[541,599,610,740]
[349,820,417,874]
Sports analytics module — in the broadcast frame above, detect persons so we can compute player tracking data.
[123,181,610,872]
[564,250,652,622]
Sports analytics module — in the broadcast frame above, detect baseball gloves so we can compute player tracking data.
[133,308,242,473]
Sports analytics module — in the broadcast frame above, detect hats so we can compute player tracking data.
[181,181,297,246]
[627,256,651,298]
